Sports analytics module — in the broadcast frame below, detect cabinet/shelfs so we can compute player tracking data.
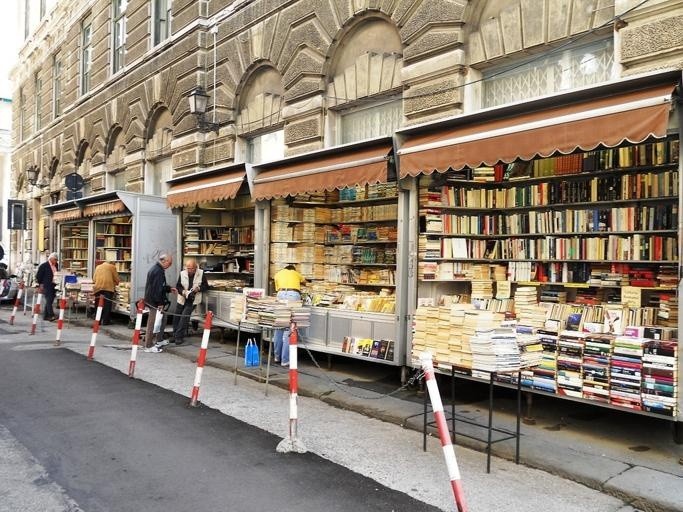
[57,218,91,278]
[94,213,132,282]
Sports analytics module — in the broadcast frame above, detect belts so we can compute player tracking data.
[279,288,300,292]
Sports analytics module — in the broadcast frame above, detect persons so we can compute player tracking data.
[36,253,60,321]
[170,259,207,344]
[143,254,176,353]
[273,265,311,367]
[93,260,120,325]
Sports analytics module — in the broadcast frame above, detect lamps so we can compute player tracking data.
[187,87,219,135]
[25,165,38,186]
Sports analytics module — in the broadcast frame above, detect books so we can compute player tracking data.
[412,140,679,417]
[343,336,395,362]
[267,182,399,314]
[180,194,255,293]
[230,291,311,329]
[64,214,132,309]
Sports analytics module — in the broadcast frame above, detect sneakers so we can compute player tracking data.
[169,337,183,344]
[274,358,289,367]
[44,315,59,322]
[144,339,169,353]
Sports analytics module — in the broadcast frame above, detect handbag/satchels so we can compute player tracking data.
[245,338,260,368]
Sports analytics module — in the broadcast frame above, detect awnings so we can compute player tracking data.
[165,169,247,208]
[399,84,678,181]
[251,143,392,202]
[52,199,126,221]
